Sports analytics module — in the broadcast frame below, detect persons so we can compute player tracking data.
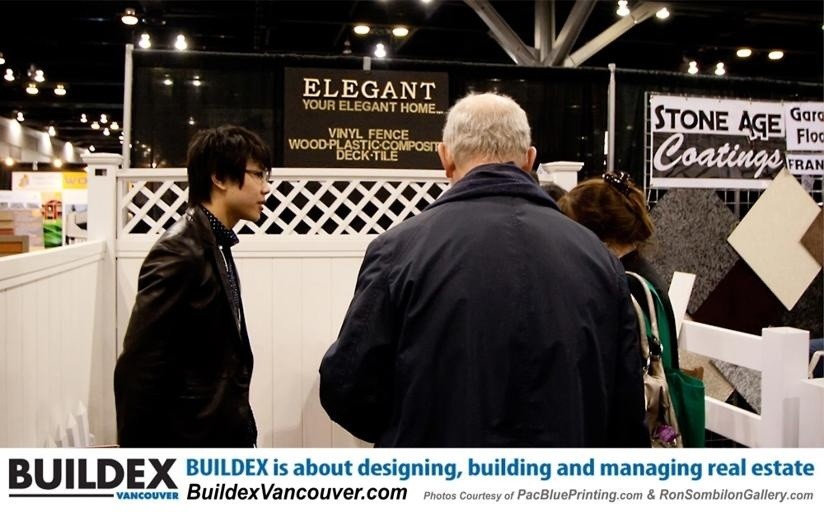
[556,170,680,446]
[110,122,271,449]
[539,182,568,204]
[320,93,653,446]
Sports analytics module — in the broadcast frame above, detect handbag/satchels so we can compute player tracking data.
[625,271,705,448]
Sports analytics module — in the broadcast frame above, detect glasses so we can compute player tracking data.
[245,168,269,184]
